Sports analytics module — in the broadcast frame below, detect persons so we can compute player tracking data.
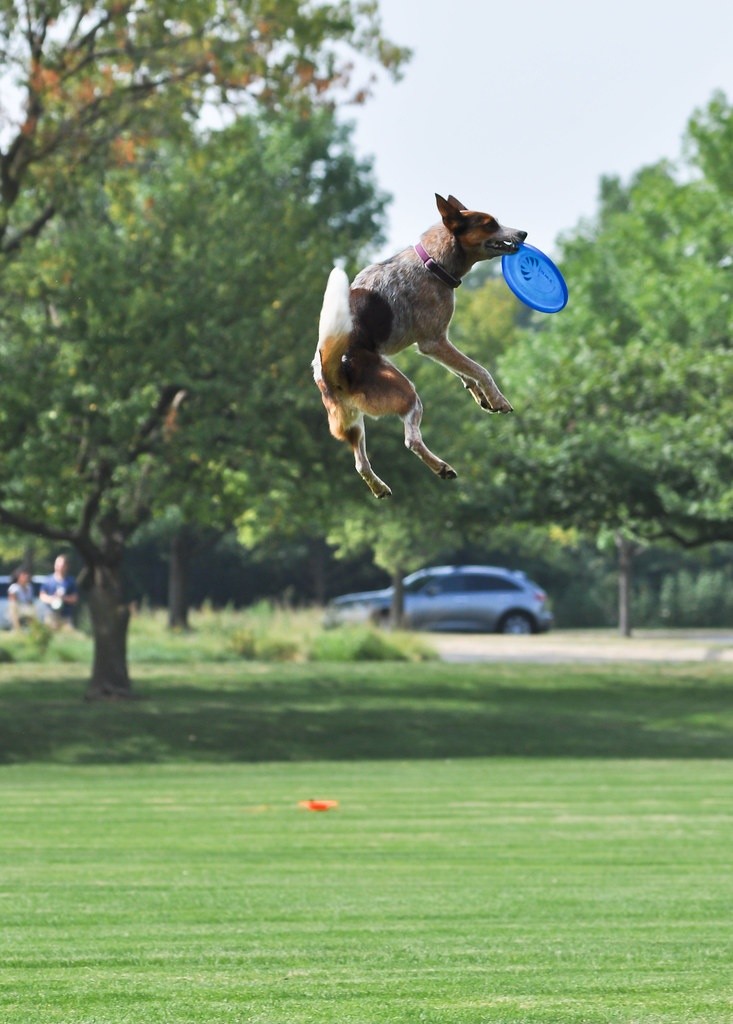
[5,565,36,629]
[40,554,80,631]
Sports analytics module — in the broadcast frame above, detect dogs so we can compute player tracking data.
[311,193,530,499]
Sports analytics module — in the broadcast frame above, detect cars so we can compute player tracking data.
[321,564,557,637]
[1,575,56,631]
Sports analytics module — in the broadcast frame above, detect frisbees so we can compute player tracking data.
[500,241,570,314]
